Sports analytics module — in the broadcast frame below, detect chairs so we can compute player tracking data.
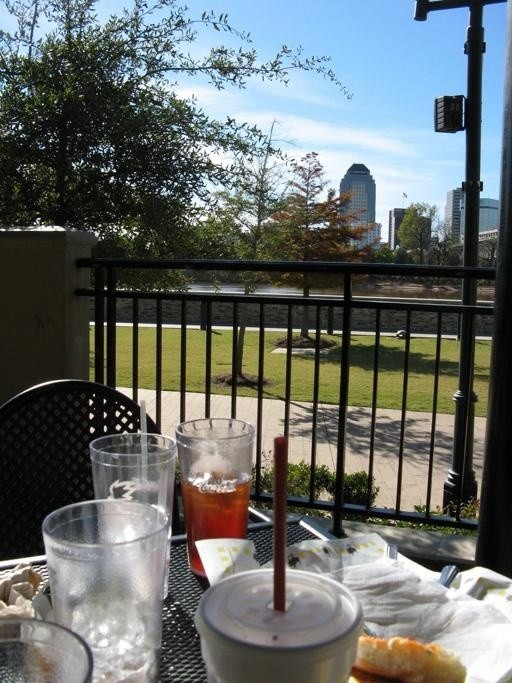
[1,376,181,565]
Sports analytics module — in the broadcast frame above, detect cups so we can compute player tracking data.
[0,617,95,683]
[88,431,176,605]
[193,568,363,683]
[175,418,256,575]
[41,496,168,682]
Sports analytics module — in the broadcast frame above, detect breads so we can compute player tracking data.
[354,634,466,683]
